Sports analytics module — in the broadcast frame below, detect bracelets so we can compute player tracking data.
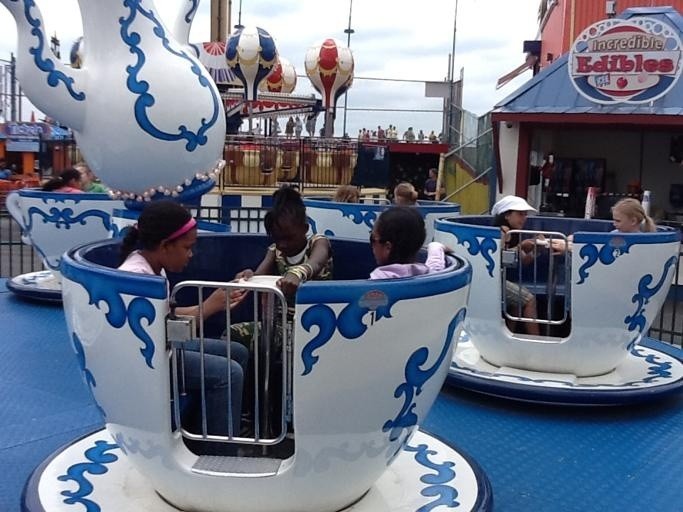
[280,262,316,283]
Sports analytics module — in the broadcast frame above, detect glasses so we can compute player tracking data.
[369,233,380,248]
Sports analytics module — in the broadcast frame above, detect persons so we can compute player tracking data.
[489,194,546,336]
[112,199,249,459]
[357,123,442,145]
[40,166,84,195]
[391,182,420,208]
[358,204,455,283]
[421,166,447,203]
[328,184,360,204]
[72,162,112,195]
[217,181,338,444]
[0,158,13,180]
[251,122,263,135]
[283,112,325,141]
[549,198,658,255]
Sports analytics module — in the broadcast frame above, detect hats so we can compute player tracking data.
[491,194,538,217]
